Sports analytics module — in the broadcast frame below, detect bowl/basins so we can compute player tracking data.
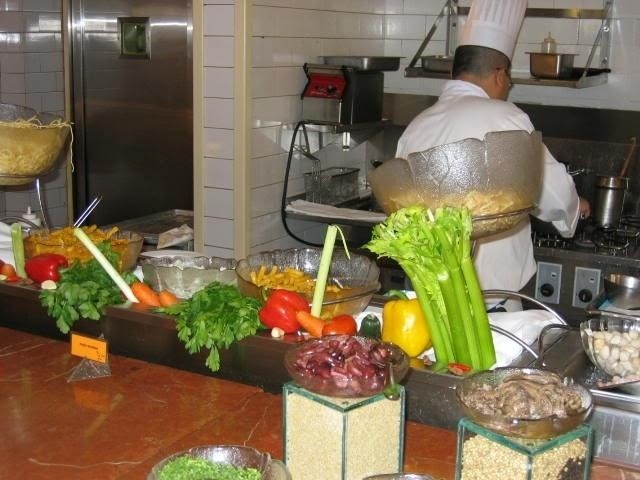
[580,318,640,376]
[285,335,409,397]
[23,227,145,275]
[0,102,73,187]
[140,254,239,302]
[604,272,640,310]
[456,366,594,441]
[367,130,544,240]
[146,444,271,480]
[235,246,379,318]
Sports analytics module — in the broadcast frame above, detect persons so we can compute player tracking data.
[394,0,591,313]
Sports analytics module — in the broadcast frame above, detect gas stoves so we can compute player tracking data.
[529,211,640,263]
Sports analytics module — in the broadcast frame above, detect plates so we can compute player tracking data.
[133,247,199,282]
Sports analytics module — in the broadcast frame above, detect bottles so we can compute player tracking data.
[541,31,556,54]
[15,205,41,233]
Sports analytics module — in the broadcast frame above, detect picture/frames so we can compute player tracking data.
[117,16,152,62]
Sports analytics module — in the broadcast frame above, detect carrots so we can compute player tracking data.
[159,289,178,307]
[130,282,159,307]
[296,310,325,339]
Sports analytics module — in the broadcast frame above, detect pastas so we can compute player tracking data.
[0,116,75,176]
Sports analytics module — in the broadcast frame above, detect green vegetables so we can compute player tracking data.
[360,204,495,377]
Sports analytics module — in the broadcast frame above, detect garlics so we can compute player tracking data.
[41,280,57,290]
[593,329,640,377]
[271,327,285,338]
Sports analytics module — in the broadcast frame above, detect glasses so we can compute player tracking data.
[496,67,514,91]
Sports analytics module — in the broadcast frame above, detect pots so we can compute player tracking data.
[561,162,634,229]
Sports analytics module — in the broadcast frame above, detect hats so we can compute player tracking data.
[456,0,528,66]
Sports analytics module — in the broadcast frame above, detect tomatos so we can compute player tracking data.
[322,315,357,337]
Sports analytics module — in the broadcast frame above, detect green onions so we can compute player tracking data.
[11,223,27,279]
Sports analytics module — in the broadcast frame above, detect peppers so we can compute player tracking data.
[24,252,78,283]
[259,289,310,334]
